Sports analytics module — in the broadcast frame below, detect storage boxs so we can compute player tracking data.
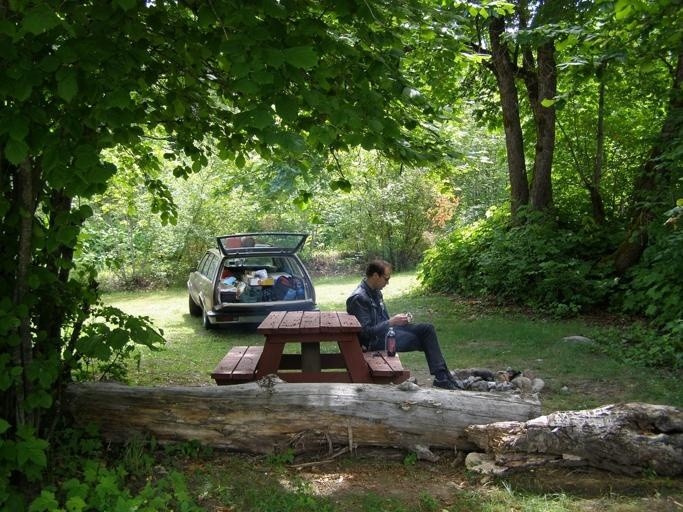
[219,285,237,302]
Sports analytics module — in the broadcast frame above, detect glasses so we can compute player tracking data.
[381,273,389,281]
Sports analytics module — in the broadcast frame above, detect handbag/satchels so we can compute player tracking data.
[237,286,262,302]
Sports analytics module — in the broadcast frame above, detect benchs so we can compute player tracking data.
[210,345,264,387]
[361,345,410,386]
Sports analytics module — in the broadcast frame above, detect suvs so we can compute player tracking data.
[187,232,320,330]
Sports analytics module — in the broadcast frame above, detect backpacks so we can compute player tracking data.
[274,276,305,300]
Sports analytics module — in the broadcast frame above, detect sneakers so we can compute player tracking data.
[433,377,465,391]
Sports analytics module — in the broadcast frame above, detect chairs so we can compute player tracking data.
[224,264,278,280]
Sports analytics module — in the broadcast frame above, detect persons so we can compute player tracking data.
[344,259,462,390]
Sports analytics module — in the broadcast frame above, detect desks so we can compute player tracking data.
[256,311,374,384]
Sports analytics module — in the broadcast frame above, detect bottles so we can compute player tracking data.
[387,327,396,357]
